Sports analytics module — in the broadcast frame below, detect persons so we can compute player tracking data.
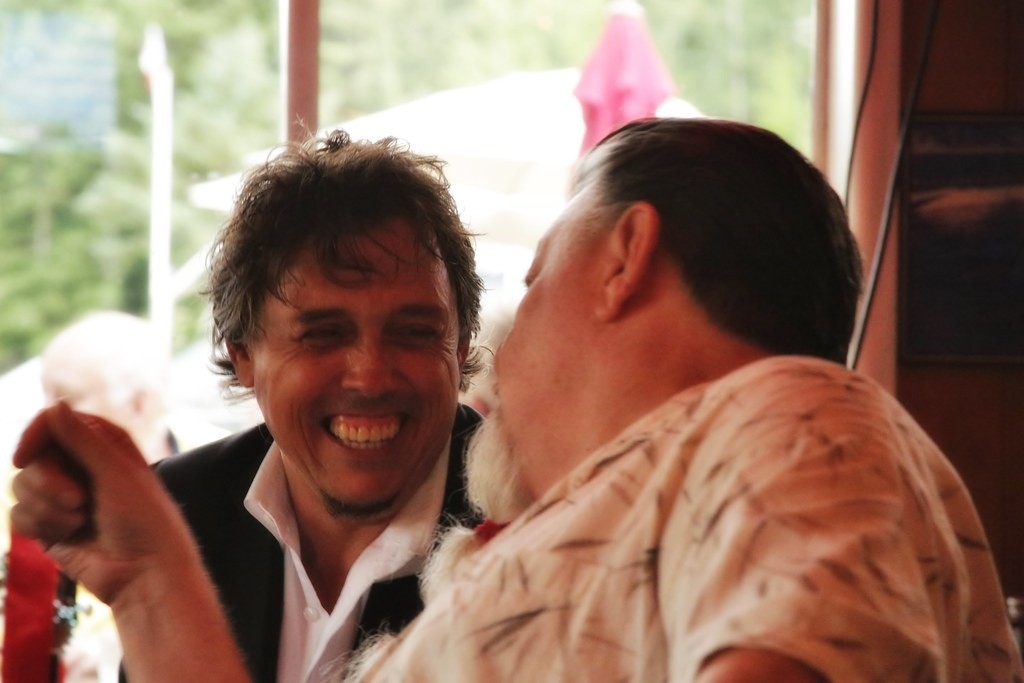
[10,118,1024,683]
